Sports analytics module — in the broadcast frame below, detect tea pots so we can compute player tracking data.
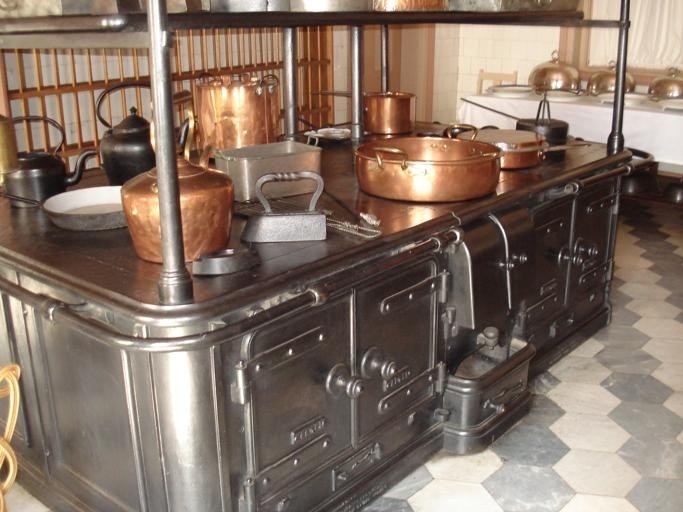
[95,80,199,185]
[113,143,236,266]
[1,115,98,211]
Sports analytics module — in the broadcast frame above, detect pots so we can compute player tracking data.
[455,128,592,170]
[361,90,418,134]
[350,122,505,203]
[189,70,282,165]
[208,0,580,17]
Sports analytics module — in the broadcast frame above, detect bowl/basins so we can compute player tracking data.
[42,186,131,231]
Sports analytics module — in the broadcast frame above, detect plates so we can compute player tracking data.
[487,83,683,115]
[304,127,352,142]
